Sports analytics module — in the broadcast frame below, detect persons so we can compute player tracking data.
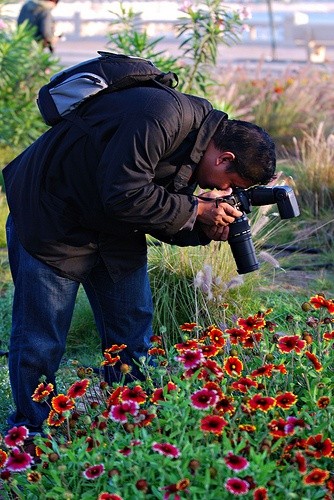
[1,87,276,465]
[15,0,61,58]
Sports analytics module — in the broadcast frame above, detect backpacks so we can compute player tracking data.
[36,51,195,163]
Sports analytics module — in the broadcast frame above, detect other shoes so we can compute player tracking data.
[25,434,44,466]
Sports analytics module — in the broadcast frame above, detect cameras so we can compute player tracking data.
[221,186,300,275]
